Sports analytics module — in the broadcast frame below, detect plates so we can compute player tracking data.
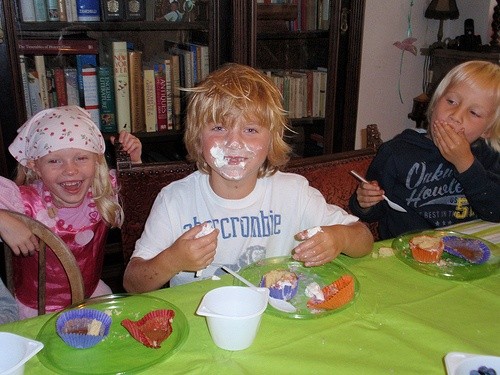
[391,229,500,281]
[444,352,500,375]
[35,293,190,375]
[234,256,361,320]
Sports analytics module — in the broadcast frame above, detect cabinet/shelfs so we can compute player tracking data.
[0,0,368,281]
[420,47,500,130]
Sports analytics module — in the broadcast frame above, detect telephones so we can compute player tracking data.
[456,19,482,52]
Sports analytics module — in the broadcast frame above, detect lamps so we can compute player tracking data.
[424,0,460,47]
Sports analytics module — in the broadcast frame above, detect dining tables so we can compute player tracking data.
[0,217,500,375]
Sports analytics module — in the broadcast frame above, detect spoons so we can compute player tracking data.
[351,170,407,212]
[221,266,297,313]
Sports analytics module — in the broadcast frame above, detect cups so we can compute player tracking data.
[0,331,44,375]
[195,286,270,351]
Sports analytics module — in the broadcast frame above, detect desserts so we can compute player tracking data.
[411,235,444,264]
[56,309,113,349]
[258,270,299,301]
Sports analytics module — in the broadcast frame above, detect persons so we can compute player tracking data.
[349,61,500,241]
[123,62,374,294]
[0,105,143,321]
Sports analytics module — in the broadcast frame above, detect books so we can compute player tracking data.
[17,0,330,140]
